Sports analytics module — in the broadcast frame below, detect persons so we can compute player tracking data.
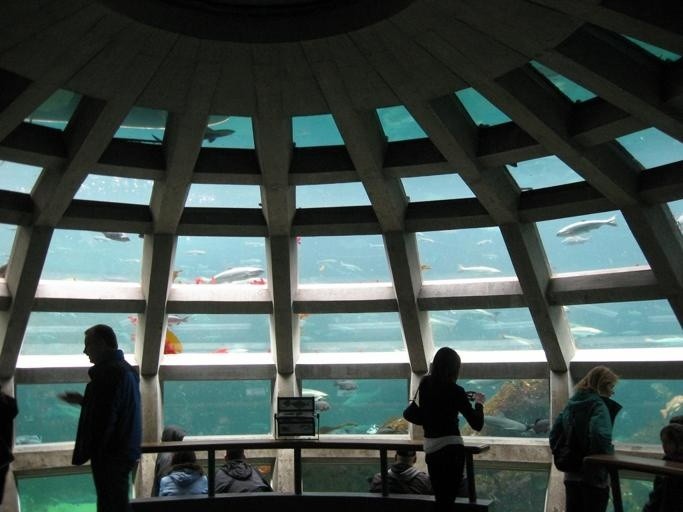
[417,347,485,510]
[58,325,143,510]
[150,426,183,496]
[640,415,682,512]
[368,447,432,495]
[156,450,207,496]
[546,364,621,512]
[214,448,274,493]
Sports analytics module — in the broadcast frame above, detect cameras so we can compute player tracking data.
[465,391,476,400]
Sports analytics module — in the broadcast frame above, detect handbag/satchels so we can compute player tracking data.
[403,402,422,426]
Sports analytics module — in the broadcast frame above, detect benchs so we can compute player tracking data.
[129,435,495,512]
[583,452,682,511]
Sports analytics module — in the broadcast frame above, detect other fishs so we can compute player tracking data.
[153,123,234,146]
[93,213,683,450]
[15,433,41,444]
